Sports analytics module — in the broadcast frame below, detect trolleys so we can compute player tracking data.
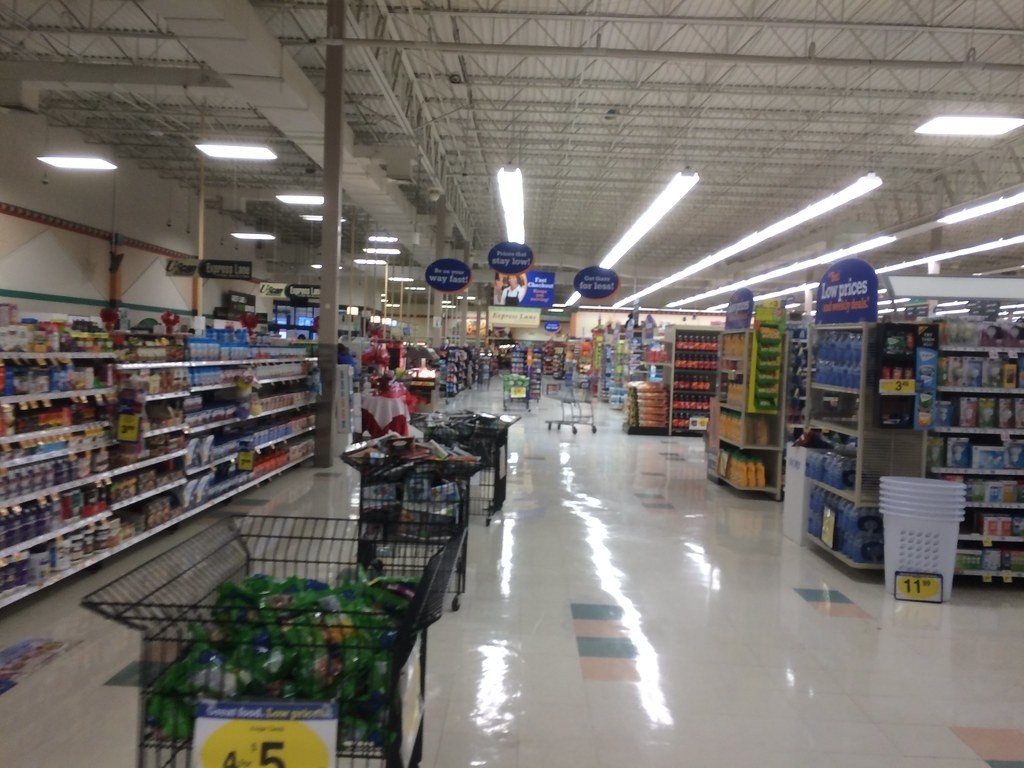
[546,377,602,435]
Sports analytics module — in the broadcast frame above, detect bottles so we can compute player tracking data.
[672,413,709,429]
[0,446,114,501]
[806,450,855,489]
[814,332,860,388]
[673,372,712,390]
[676,354,717,370]
[807,485,884,563]
[187,327,252,359]
[68,520,110,564]
[37,316,113,352]
[673,393,711,412]
[677,335,716,351]
[0,492,61,550]
[188,367,224,385]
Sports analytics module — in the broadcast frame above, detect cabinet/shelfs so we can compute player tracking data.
[716,328,789,501]
[807,319,938,570]
[0,351,316,608]
[939,322,1024,583]
[663,324,725,437]
